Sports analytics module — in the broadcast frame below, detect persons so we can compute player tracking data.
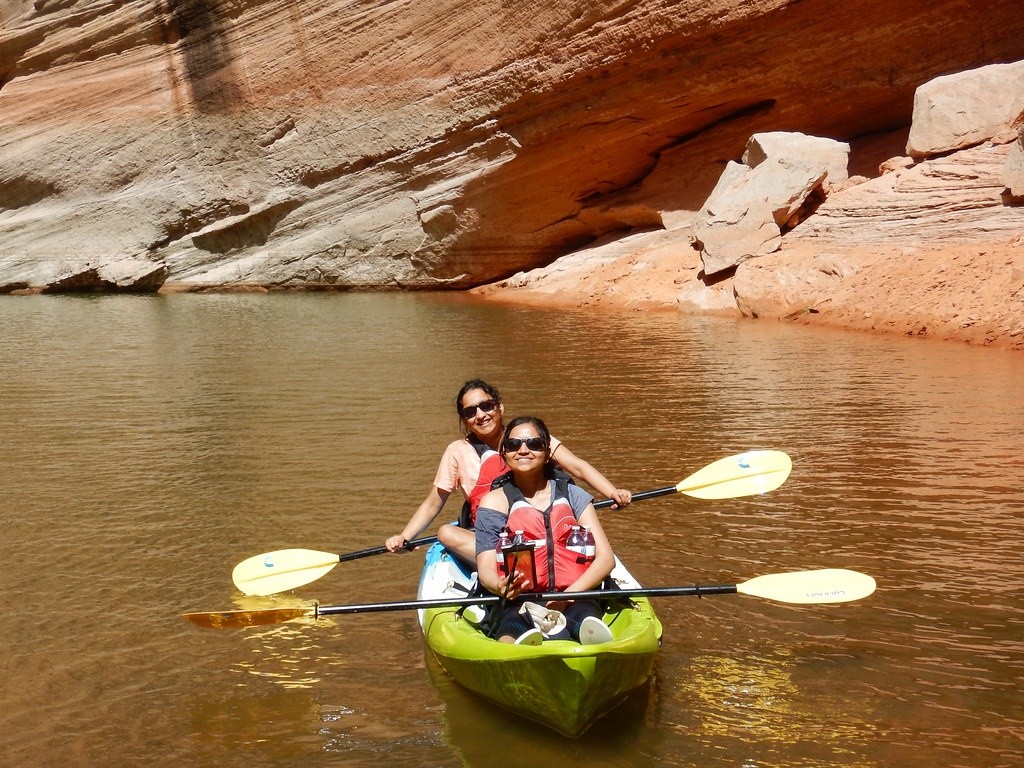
[385,378,630,567]
[474,414,615,645]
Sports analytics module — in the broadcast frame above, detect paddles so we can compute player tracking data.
[179,565,878,630]
[230,448,793,596]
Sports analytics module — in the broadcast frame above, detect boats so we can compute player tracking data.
[413,516,665,741]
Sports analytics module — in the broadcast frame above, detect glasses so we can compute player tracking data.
[461,399,497,419]
[504,437,546,452]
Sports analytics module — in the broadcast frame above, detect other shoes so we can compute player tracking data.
[514,628,543,645]
[578,616,612,645]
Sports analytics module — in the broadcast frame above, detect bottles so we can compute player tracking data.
[496,532,512,562]
[582,526,595,556]
[513,530,527,544]
[565,526,586,555]
